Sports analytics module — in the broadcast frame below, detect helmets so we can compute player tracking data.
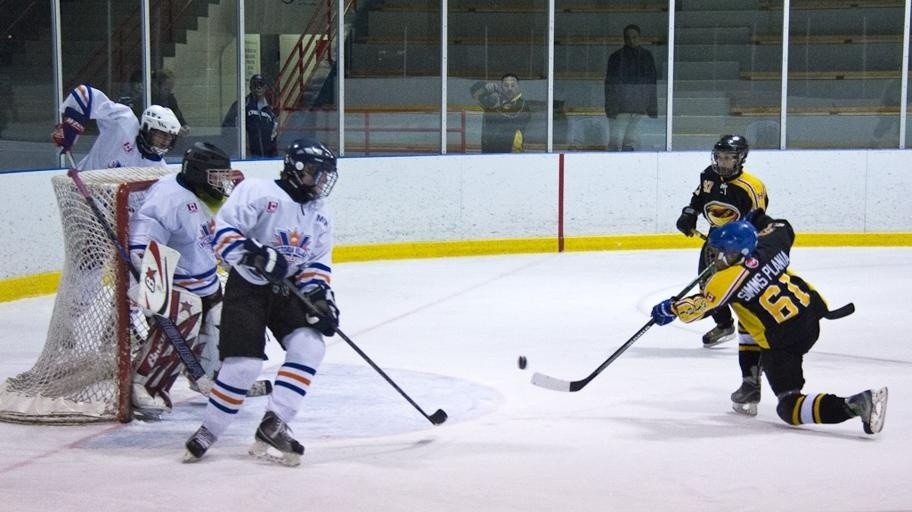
[281,139,338,202]
[183,143,232,201]
[707,222,759,266]
[140,104,183,157]
[711,135,749,181]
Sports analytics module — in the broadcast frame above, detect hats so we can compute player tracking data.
[251,74,266,84]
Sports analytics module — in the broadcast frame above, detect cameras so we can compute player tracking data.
[476,90,507,112]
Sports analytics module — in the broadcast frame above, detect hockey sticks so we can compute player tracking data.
[69,168,273,400]
[517,261,720,394]
[282,276,450,426]
[692,228,856,321]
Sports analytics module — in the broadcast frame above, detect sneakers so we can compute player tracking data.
[130,371,173,413]
[254,410,307,457]
[731,380,760,405]
[701,319,737,344]
[185,424,216,459]
[845,388,875,435]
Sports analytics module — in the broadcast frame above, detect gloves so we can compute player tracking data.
[675,206,699,238]
[745,208,757,224]
[238,238,288,287]
[650,296,676,327]
[52,118,86,155]
[304,286,340,337]
[127,238,181,318]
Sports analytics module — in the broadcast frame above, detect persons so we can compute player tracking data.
[651,208,888,436]
[471,74,531,153]
[220,74,279,158]
[180,136,340,466]
[676,133,770,348]
[51,81,180,347]
[118,70,143,123]
[130,142,273,419]
[153,70,191,135]
[603,24,658,153]
